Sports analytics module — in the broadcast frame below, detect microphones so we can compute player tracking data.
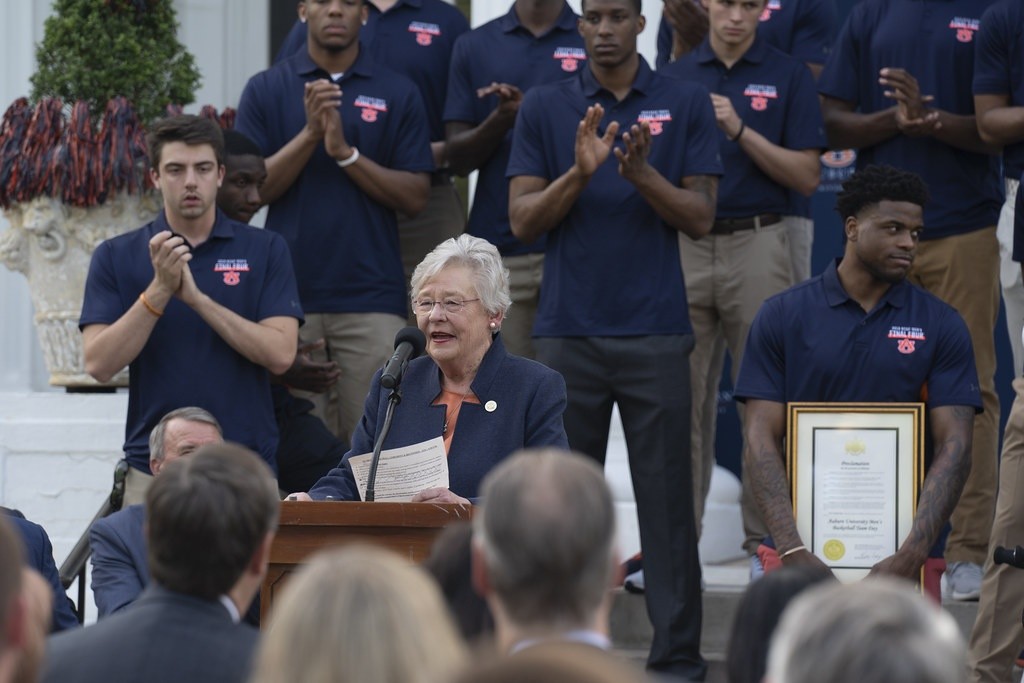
[381,327,427,388]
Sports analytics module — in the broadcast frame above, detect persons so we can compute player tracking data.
[0,506,81,683]
[731,164,986,592]
[443,0,585,361]
[284,232,570,507]
[245,537,474,683]
[505,0,725,683]
[78,113,304,515]
[86,405,261,634]
[619,0,1024,683]
[759,571,982,683]
[271,0,472,361]
[232,0,437,460]
[35,441,283,683]
[423,446,690,683]
[214,128,344,497]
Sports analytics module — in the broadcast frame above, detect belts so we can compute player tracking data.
[709,213,782,235]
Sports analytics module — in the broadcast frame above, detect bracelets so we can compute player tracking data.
[727,118,745,145]
[778,545,807,560]
[335,146,360,168]
[139,293,164,317]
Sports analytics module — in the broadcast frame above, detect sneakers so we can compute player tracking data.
[945,559,983,601]
[624,569,706,594]
[749,554,763,585]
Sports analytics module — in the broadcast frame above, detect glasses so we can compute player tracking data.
[412,293,480,316]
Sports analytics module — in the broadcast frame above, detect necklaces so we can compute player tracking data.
[438,367,476,433]
